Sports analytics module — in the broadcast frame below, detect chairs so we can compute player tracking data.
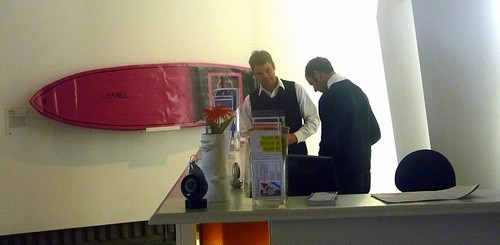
[395,148,456,192]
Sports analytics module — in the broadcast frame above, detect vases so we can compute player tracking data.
[202,133,230,202]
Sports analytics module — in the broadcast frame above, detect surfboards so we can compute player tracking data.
[29,63,260,133]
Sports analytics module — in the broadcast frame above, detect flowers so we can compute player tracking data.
[202,104,236,135]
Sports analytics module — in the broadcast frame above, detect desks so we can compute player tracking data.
[148,140,500,245]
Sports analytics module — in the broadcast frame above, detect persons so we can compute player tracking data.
[305,57,381,194]
[240,50,320,155]
